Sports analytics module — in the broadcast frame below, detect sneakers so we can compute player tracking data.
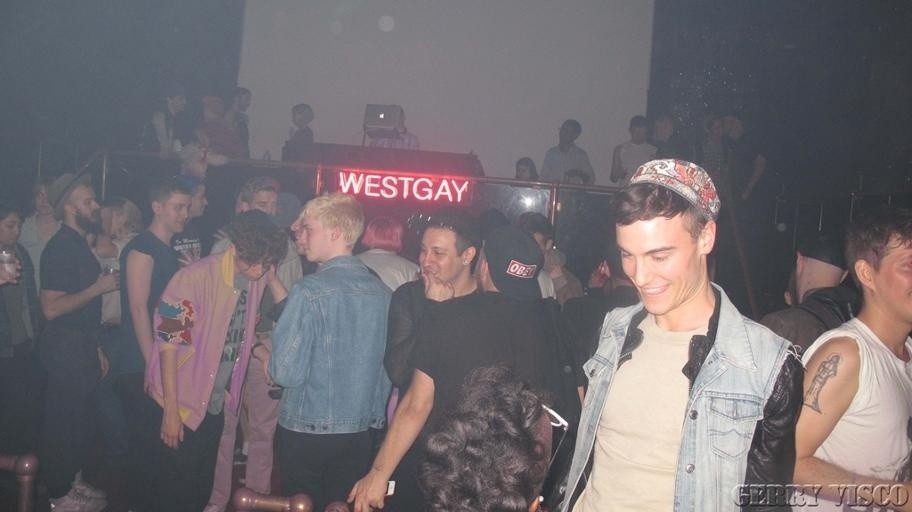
[49,472,108,512]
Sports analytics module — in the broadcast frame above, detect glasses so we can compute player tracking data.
[541,403,570,471]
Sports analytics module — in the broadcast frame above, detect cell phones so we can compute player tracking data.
[384,480,396,496]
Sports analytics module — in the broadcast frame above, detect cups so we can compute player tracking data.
[1,245,19,280]
[104,265,122,279]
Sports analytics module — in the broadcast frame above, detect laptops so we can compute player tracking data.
[364,104,402,129]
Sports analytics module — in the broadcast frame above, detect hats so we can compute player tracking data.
[629,157,720,222]
[48,175,95,220]
[798,225,847,271]
[486,224,543,302]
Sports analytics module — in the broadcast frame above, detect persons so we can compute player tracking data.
[0,86,912,512]
